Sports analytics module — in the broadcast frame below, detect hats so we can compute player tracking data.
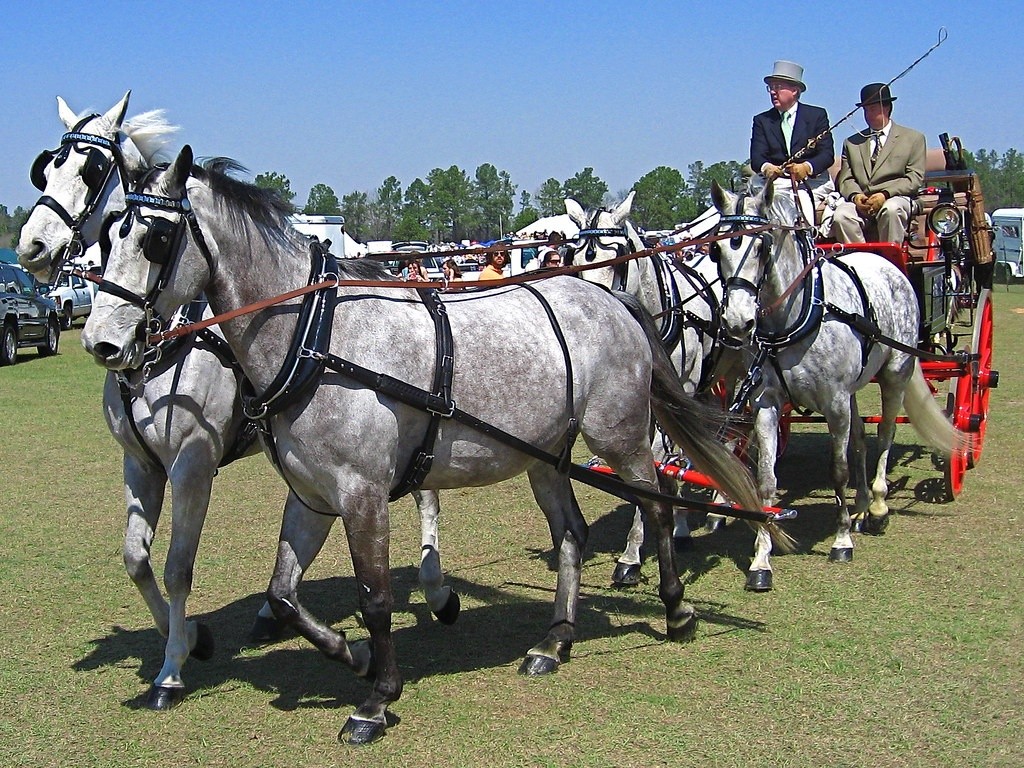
[855,83,897,106]
[764,60,806,92]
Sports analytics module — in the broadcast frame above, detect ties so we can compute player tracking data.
[781,111,792,157]
[870,131,884,170]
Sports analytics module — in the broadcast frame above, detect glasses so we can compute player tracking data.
[550,260,561,264]
[494,252,506,256]
[766,84,794,92]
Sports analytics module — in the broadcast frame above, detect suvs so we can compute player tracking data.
[0,262,62,367]
[23,263,103,332]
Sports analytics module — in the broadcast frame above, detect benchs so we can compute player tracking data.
[815,192,968,260]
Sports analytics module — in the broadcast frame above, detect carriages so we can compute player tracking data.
[11,87,1001,746]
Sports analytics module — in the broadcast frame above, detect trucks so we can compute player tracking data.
[988,206,1024,284]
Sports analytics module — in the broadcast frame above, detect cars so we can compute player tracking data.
[284,213,578,284]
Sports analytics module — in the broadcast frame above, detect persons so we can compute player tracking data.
[830,83,927,245]
[396,226,577,293]
[749,60,835,233]
[88,260,101,275]
[695,241,709,258]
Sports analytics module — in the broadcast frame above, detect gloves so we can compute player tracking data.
[762,163,786,182]
[864,192,886,217]
[854,194,874,222]
[786,162,811,181]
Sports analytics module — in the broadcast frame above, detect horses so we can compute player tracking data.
[15,89,978,743]
